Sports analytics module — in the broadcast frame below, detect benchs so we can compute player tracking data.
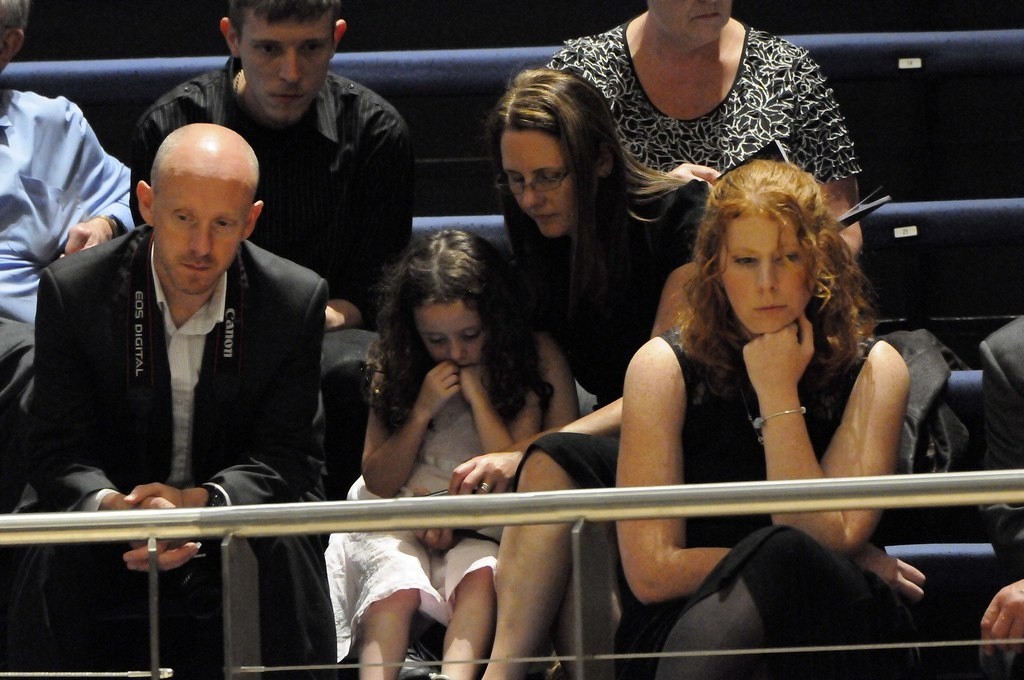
[0,30,1024,680]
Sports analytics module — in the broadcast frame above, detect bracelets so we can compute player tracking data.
[94,215,119,239]
[206,485,225,508]
[753,407,807,428]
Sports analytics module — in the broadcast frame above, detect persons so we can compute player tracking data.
[449,68,714,680]
[324,229,553,680]
[546,0,864,257]
[4,123,337,680]
[978,314,1024,680]
[130,0,413,329]
[0,0,137,460]
[612,160,927,680]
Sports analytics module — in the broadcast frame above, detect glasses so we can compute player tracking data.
[493,168,568,196]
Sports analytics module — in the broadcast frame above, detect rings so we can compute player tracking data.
[482,483,493,492]
